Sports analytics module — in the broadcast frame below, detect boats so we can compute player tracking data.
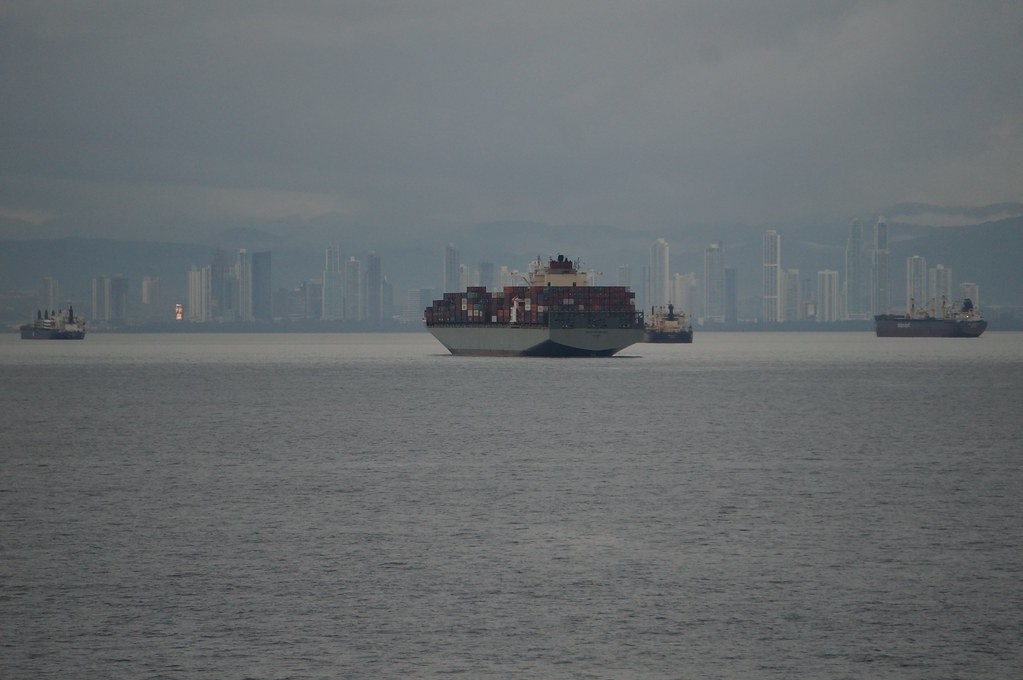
[874,294,989,337]
[19,306,85,340]
[647,304,694,344]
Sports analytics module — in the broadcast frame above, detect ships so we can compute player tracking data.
[423,255,646,357]
